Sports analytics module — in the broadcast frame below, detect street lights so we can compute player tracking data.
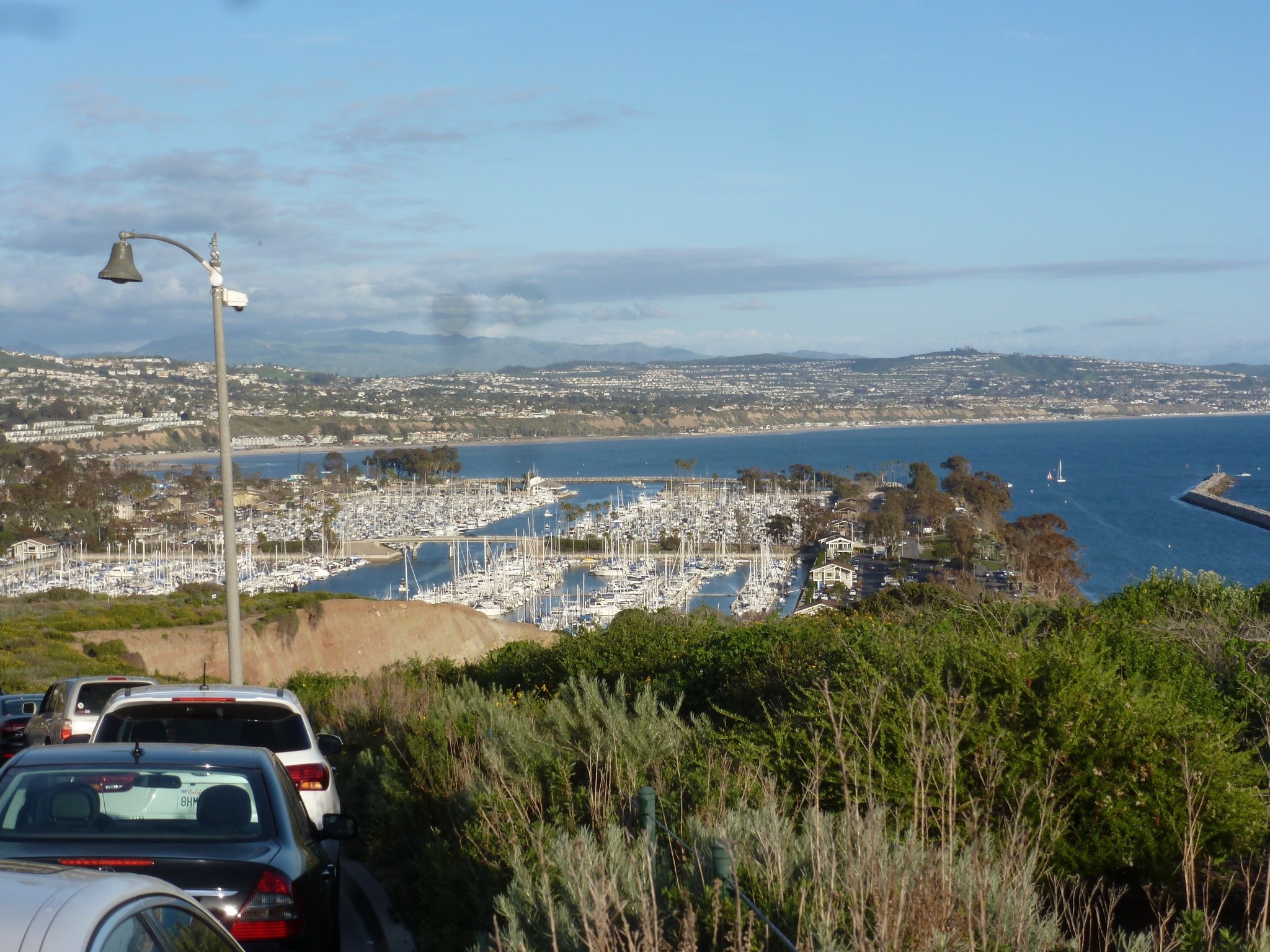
[99,225,247,689]
[913,521,919,552]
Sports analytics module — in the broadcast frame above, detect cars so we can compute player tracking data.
[853,579,858,583]
[983,572,990,577]
[854,558,859,563]
[830,594,837,600]
[847,595,854,601]
[0,694,47,761]
[985,578,991,583]
[868,564,875,570]
[0,740,361,952]
[850,590,856,596]
[17,675,159,751]
[0,851,248,952]
[852,572,858,580]
[898,540,907,547]
[853,565,859,571]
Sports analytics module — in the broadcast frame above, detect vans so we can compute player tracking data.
[821,594,828,601]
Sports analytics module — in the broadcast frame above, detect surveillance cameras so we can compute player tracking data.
[222,287,248,312]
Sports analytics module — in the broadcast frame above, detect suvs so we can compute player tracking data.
[89,679,351,872]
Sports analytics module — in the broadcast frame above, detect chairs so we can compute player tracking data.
[44,785,125,832]
[195,785,254,827]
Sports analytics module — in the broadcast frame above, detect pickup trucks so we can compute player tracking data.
[812,593,820,600]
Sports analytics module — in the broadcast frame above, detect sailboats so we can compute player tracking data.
[0,464,885,641]
[1047,470,1053,481]
[1056,459,1066,482]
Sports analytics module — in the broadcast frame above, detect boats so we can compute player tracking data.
[1004,483,1013,489]
